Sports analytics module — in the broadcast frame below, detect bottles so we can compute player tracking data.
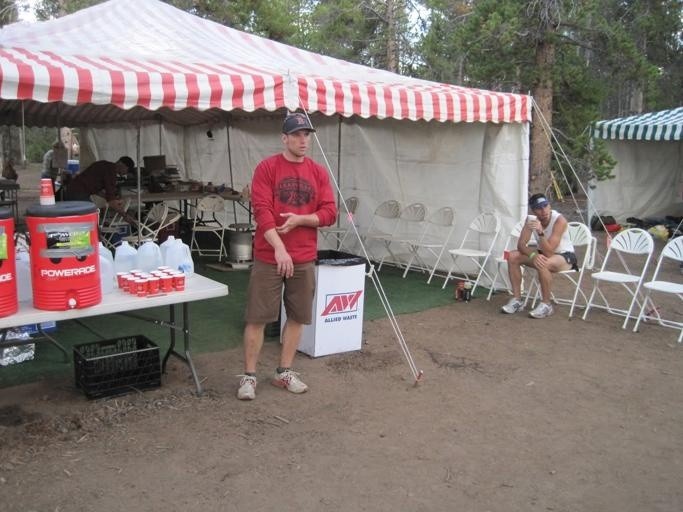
[187,179,213,193]
[454,278,471,301]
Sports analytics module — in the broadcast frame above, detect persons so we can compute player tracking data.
[2,158,18,183]
[235,112,341,402]
[64,155,139,229]
[498,192,578,320]
[40,141,66,196]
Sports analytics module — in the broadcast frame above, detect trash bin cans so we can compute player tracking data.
[280,250,366,359]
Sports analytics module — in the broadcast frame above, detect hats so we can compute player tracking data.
[281,112,316,135]
[529,194,548,209]
[120,157,135,176]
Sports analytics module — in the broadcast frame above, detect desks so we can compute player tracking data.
[0,273,229,394]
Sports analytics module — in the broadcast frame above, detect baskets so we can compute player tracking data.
[72,335,161,401]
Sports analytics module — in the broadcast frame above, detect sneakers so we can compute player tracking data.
[272,368,309,393]
[528,302,554,319]
[235,375,256,400]
[501,298,524,314]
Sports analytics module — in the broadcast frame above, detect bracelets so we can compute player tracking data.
[537,231,545,237]
[528,252,536,259]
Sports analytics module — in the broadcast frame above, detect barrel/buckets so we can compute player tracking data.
[114,240,139,290]
[16,246,34,303]
[160,235,176,265]
[0,206,20,318]
[98,241,114,295]
[137,238,163,274]
[23,200,102,310]
[166,239,195,278]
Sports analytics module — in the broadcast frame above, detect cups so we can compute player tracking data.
[503,249,510,259]
[38,177,55,206]
[527,214,537,223]
[116,266,185,297]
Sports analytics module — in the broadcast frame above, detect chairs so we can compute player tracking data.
[0,177,254,272]
